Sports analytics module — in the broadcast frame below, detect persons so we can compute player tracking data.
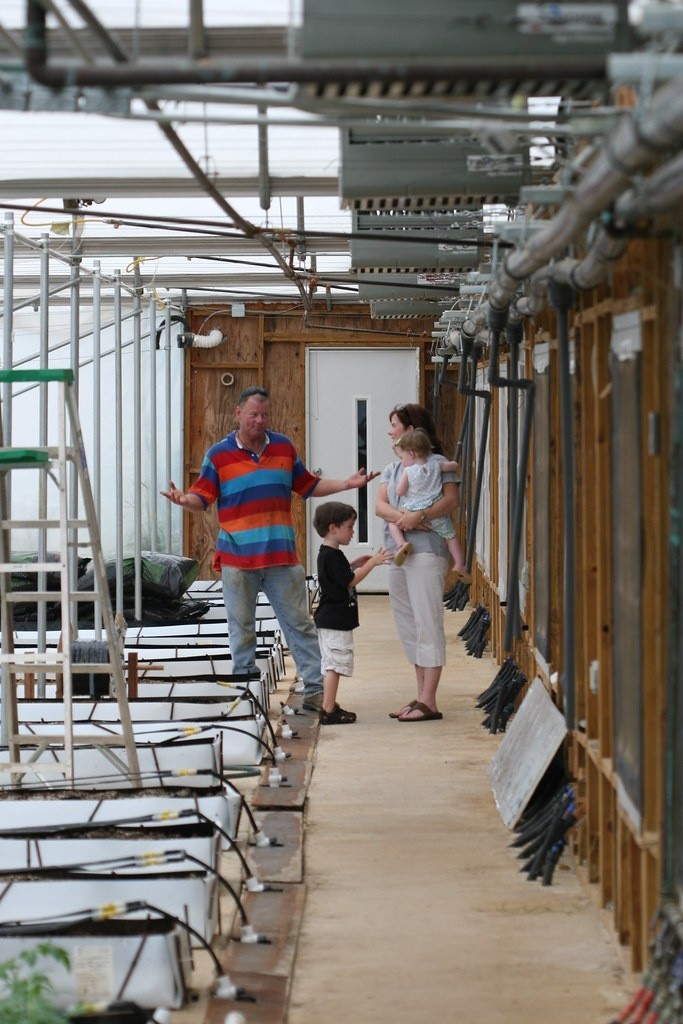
[312,501,396,723]
[387,428,473,587]
[160,386,382,710]
[376,405,463,721]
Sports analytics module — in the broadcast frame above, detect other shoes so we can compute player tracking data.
[301,693,340,712]
[393,541,412,567]
[451,568,472,584]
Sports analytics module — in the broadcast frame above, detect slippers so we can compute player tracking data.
[389,699,417,718]
[398,701,443,721]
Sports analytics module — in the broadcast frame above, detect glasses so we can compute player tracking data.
[395,404,412,424]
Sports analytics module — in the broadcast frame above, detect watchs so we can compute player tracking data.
[421,508,428,524]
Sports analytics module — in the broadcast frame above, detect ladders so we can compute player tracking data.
[0,370,144,800]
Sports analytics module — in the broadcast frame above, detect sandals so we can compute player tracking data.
[317,707,356,725]
